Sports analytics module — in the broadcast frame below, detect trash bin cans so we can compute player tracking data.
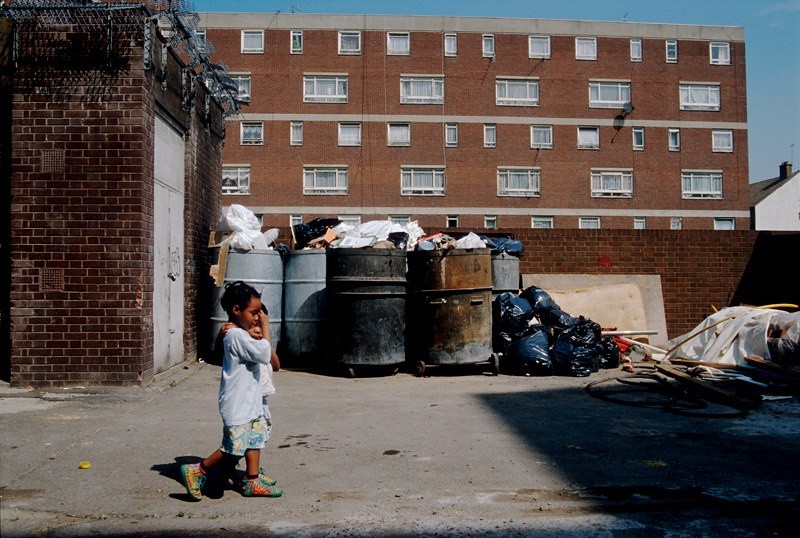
[207,200,283,361]
[485,237,524,309]
[324,219,412,376]
[413,229,501,378]
[283,216,354,362]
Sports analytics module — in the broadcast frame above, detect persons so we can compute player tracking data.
[215,303,281,486]
[181,280,284,503]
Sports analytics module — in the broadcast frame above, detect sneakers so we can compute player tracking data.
[242,476,282,497]
[244,473,277,486]
[181,462,204,501]
